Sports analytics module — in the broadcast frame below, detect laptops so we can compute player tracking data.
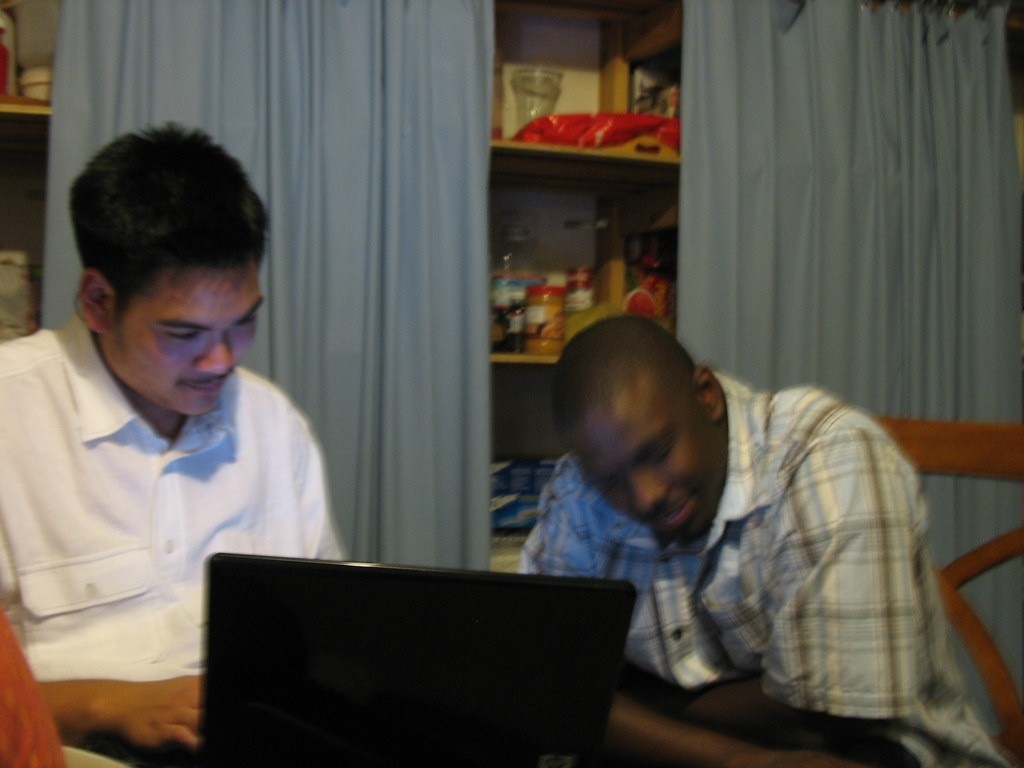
[200,553,637,768]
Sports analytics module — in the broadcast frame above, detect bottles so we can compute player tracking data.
[506,300,527,354]
[489,224,542,311]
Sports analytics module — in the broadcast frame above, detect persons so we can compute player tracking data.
[0,118,354,766]
[517,316,1012,768]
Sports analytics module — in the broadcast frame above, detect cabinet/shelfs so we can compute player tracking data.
[491,0,684,573]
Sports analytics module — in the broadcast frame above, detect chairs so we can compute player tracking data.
[874,415,1024,767]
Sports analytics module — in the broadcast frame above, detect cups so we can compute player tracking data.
[511,69,562,129]
